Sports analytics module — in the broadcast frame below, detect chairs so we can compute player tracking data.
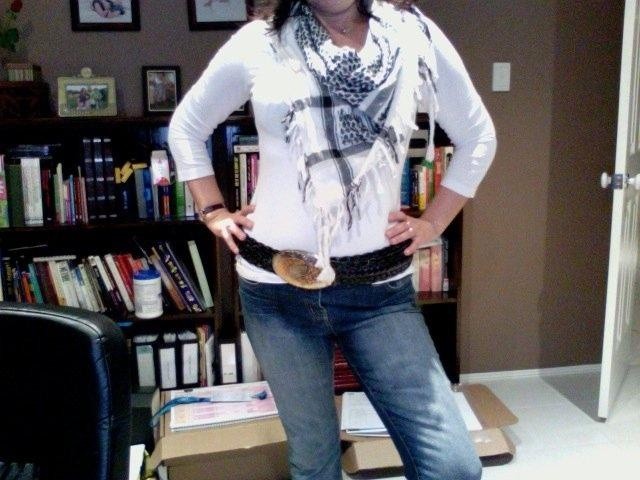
[0,302,133,479]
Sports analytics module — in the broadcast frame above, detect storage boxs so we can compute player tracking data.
[144,381,292,480]
[333,381,522,475]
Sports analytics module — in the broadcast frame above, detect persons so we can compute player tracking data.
[76,88,90,113]
[86,88,97,110]
[93,87,106,110]
[164,0,503,480]
[90,0,126,20]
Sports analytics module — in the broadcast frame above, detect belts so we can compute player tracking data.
[232,230,416,293]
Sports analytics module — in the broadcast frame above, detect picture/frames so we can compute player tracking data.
[141,65,182,117]
[69,0,142,32]
[57,67,119,119]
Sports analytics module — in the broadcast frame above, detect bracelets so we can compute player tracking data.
[193,203,230,222]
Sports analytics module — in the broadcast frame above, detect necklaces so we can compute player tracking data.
[320,12,353,36]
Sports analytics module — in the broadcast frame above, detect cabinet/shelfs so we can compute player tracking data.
[220,115,466,394]
[187,0,255,31]
[0,112,227,409]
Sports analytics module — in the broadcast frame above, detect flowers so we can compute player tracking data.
[1,0,26,58]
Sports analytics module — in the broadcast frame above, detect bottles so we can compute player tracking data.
[151,151,173,220]
[133,270,165,320]
[157,177,173,220]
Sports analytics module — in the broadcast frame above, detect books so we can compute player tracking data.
[169,381,277,430]
[341,392,389,437]
